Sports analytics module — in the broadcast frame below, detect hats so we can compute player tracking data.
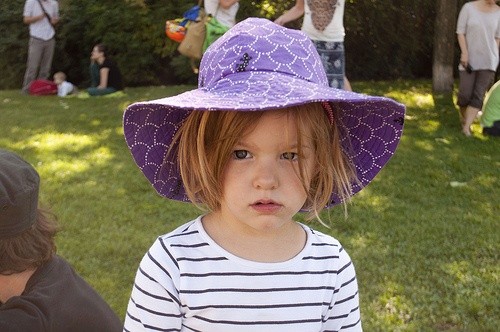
[123,18,406,213]
[0,150,41,238]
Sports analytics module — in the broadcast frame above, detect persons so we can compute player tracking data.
[202,0,353,91]
[86,44,125,96]
[22,0,61,89]
[122,17,363,332]
[53,72,79,97]
[0,147,123,332]
[456,0,500,137]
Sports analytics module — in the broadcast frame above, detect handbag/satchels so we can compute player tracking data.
[165,16,230,59]
